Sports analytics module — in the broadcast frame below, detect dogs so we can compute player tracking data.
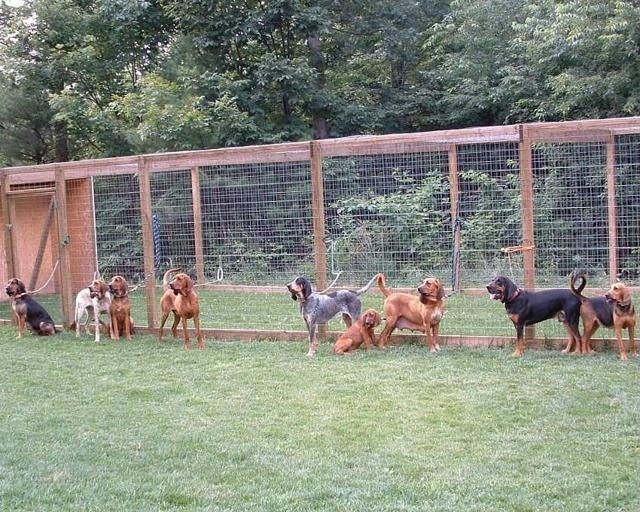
[485,275,587,358]
[333,308,378,356]
[74,279,115,342]
[158,268,206,352]
[377,273,445,354]
[569,272,640,362]
[107,275,136,341]
[286,273,379,357]
[4,277,65,340]
